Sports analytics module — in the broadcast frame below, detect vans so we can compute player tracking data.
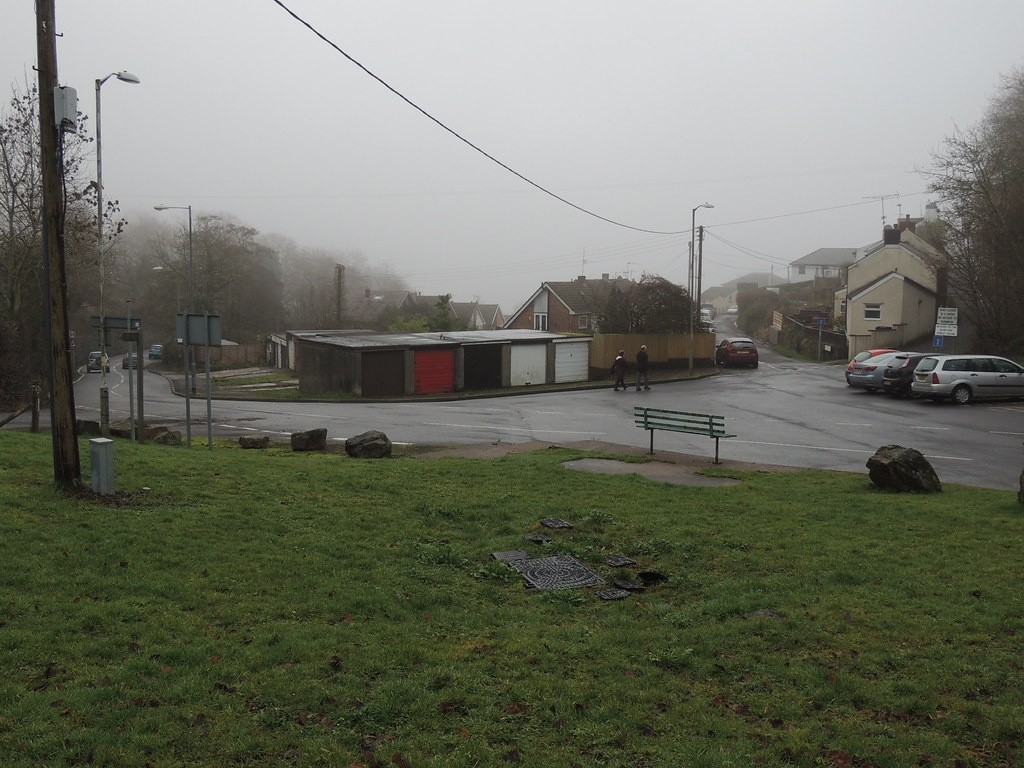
[912,355,1024,404]
[149,345,163,359]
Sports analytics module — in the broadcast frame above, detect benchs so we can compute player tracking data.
[633,404,738,465]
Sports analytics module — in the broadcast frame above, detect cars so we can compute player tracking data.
[715,338,759,368]
[845,349,902,386]
[698,310,716,341]
[882,353,948,400]
[849,352,920,393]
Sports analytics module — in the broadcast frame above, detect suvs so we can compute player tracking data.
[122,353,144,369]
[87,352,111,374]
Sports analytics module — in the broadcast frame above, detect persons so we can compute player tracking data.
[612,350,629,391]
[635,344,651,391]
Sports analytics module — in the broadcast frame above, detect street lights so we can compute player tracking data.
[154,204,197,395]
[94,69,140,438]
[153,267,182,359]
[689,201,714,376]
